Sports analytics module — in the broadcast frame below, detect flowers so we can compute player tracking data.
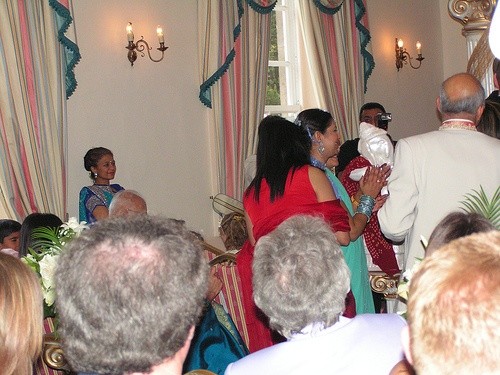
[22,214,93,338]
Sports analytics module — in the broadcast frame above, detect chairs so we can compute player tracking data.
[210,251,273,353]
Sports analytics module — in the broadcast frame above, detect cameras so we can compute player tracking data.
[376,113,392,130]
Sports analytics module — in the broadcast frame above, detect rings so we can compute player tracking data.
[377,180,380,183]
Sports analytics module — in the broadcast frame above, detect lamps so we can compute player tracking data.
[395,36,424,73]
[124,19,169,67]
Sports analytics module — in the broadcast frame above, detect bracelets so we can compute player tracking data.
[357,194,375,223]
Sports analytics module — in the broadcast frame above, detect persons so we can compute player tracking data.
[53,215,209,375]
[293,102,397,316]
[78,147,250,375]
[476,58,500,140]
[225,214,408,375]
[242,115,356,318]
[0,213,65,375]
[425,212,496,257]
[391,230,500,375]
[377,72,500,316]
